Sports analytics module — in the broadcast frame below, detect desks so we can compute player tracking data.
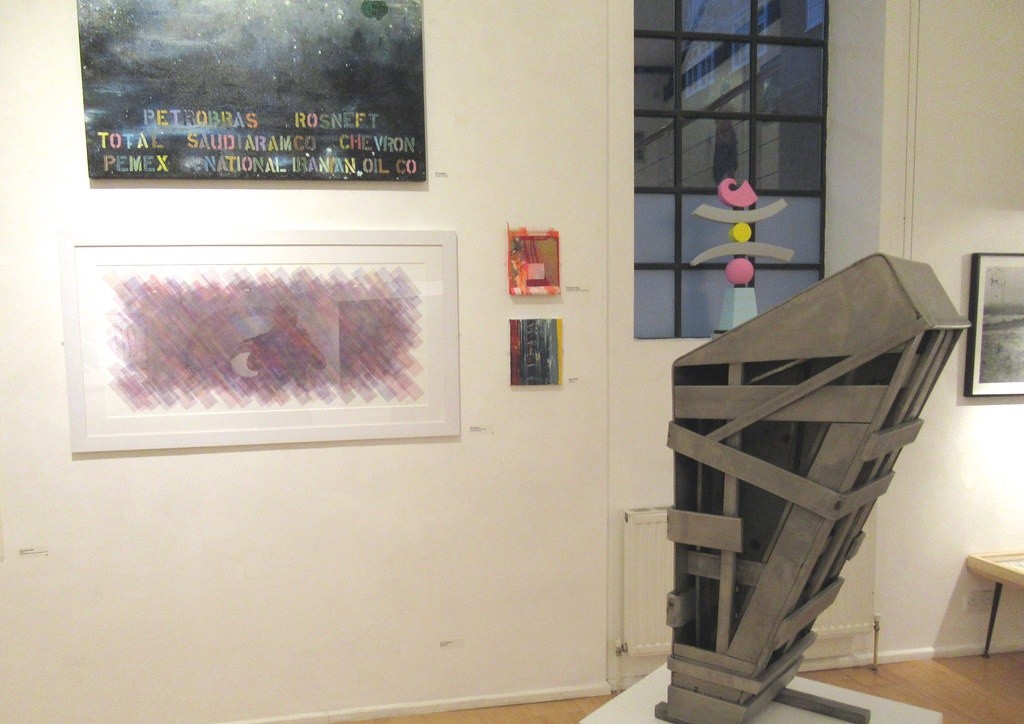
[968,551,1024,659]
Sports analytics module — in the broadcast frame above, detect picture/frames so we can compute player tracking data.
[56,230,463,453]
[964,253,1024,396]
